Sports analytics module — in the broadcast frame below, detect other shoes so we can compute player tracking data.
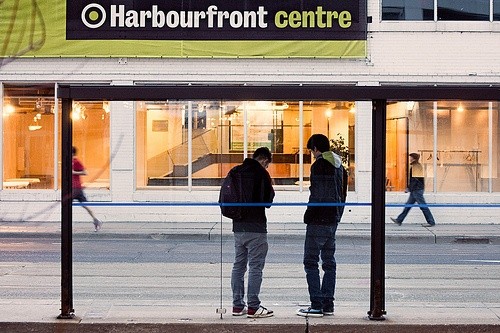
[390,218,402,226]
[93,219,102,232]
[423,223,435,227]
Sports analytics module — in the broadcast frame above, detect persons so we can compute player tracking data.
[296,134,349,318]
[390,153,435,227]
[72,146,103,232]
[227,147,275,318]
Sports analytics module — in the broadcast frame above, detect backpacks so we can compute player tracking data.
[219,165,262,220]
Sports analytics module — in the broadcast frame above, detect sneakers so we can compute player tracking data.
[322,307,335,315]
[247,306,274,318]
[232,304,248,316]
[296,308,323,318]
